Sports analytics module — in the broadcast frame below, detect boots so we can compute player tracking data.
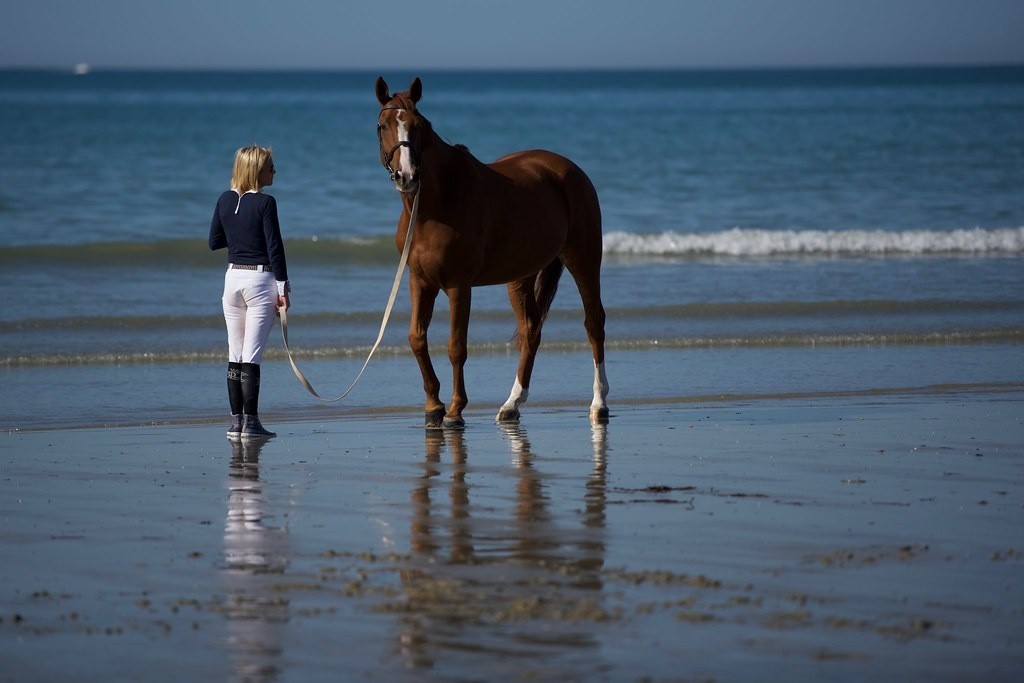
[227,412,277,437]
[228,437,270,467]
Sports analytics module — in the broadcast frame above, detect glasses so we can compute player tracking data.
[266,165,275,175]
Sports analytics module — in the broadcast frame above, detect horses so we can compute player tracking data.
[375,77,609,429]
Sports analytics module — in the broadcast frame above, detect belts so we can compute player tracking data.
[227,264,272,272]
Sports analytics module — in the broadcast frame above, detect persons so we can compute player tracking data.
[224,435,285,681]
[209,144,290,440]
